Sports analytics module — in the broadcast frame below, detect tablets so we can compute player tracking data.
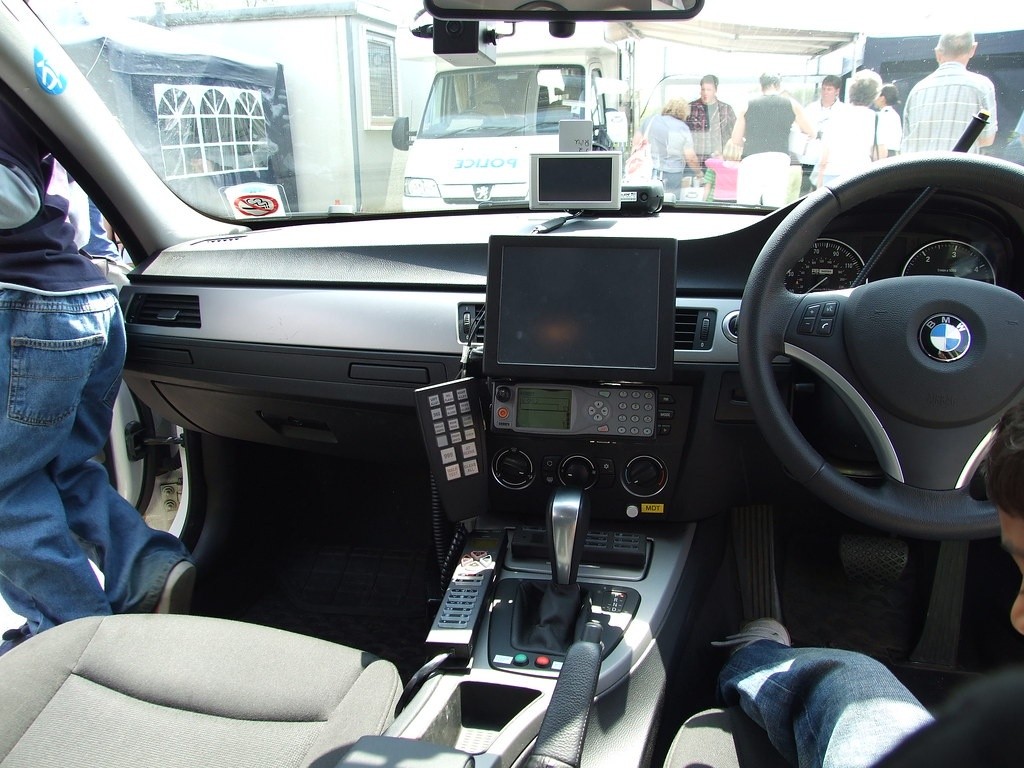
[530,150,624,209]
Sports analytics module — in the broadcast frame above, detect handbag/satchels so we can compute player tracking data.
[624,139,652,180]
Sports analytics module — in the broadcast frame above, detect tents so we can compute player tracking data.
[49,11,302,218]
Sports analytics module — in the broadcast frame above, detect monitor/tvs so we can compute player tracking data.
[482,232,679,384]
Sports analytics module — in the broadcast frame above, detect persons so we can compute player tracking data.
[808,72,882,191]
[790,75,846,197]
[898,31,1000,161]
[730,72,816,208]
[712,398,1024,768]
[870,83,903,166]
[0,106,199,639]
[1004,105,1024,166]
[641,93,704,203]
[686,74,737,178]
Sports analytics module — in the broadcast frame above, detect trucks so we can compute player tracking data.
[389,42,668,212]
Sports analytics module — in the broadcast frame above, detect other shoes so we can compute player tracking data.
[154,558,196,615]
[0,621,32,657]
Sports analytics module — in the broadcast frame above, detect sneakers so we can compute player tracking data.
[711,615,790,658]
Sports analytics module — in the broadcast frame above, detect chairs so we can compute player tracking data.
[0,613,402,768]
[661,707,790,768]
